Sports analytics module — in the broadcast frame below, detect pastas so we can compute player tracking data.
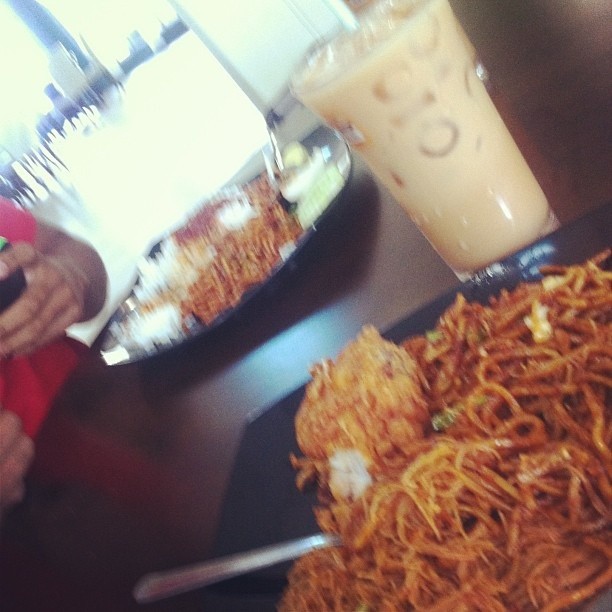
[274,246,611,612]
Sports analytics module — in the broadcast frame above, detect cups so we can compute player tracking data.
[289,0,562,286]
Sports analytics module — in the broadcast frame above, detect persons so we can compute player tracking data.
[1,193,110,513]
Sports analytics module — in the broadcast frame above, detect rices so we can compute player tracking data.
[116,174,302,349]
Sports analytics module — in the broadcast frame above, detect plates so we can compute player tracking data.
[202,204,611,612]
[100,122,354,370]
